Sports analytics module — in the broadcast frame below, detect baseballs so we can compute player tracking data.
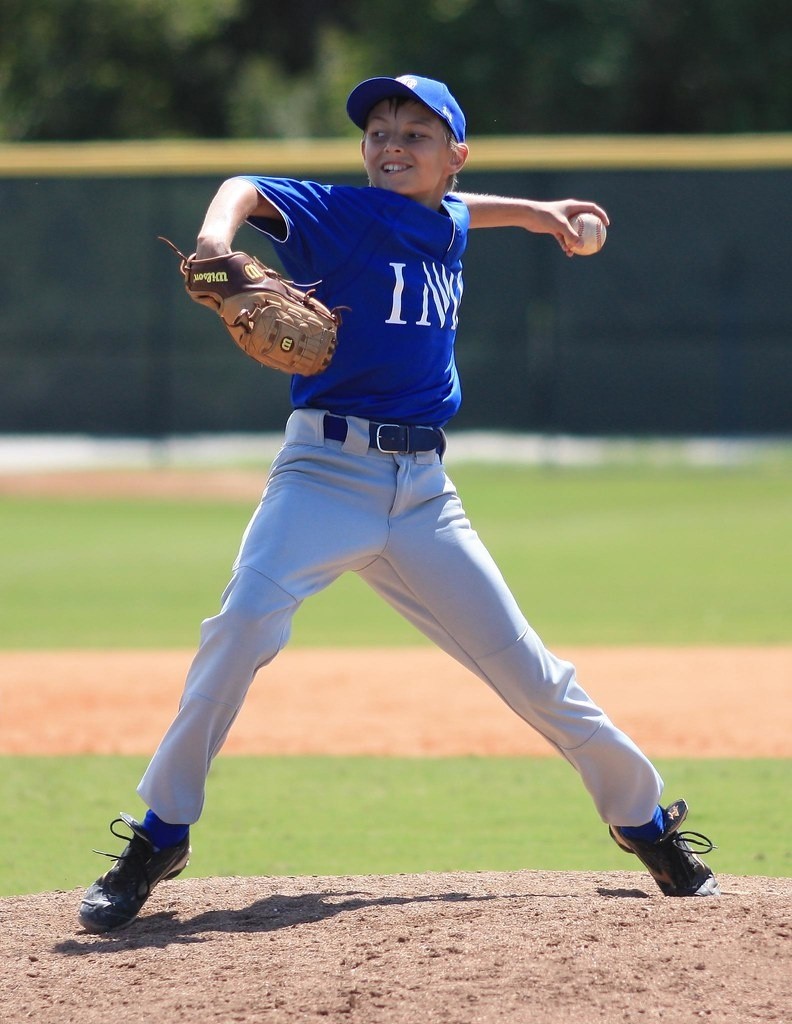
[567,211,606,256]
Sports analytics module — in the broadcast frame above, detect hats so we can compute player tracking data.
[347,74,466,142]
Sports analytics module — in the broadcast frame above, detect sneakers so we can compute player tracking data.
[76,813,191,932]
[609,799,720,896]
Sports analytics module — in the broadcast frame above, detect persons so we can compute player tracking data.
[77,73,721,933]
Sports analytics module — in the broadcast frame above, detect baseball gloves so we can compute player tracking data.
[155,234,360,380]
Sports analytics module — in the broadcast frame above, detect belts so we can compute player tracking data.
[323,415,440,454]
